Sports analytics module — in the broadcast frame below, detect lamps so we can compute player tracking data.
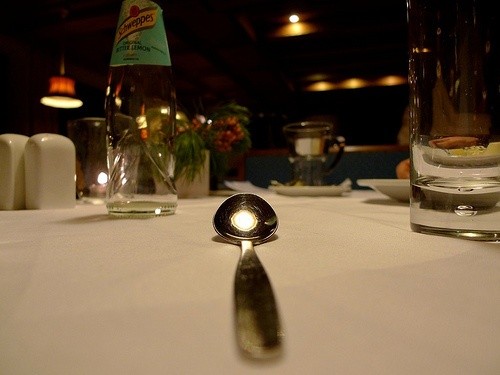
[40,51,84,110]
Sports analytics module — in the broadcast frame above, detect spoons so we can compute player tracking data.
[212,192,282,361]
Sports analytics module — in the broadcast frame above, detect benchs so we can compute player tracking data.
[237,144,410,186]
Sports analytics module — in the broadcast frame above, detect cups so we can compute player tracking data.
[68,118,107,204]
[282,122,347,187]
[407,0,500,242]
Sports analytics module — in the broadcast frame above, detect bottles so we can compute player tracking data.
[104,0,177,216]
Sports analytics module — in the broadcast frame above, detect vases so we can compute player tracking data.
[172,148,210,197]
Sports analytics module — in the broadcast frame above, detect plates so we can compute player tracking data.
[358,178,409,204]
[268,185,351,197]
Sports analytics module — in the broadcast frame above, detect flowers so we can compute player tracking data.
[172,97,251,190]
